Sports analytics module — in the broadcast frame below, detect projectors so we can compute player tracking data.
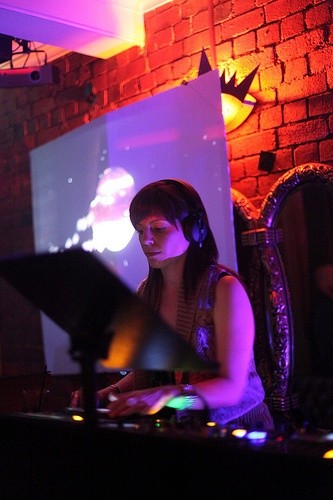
[0,66,59,88]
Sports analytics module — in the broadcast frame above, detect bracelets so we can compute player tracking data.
[111,384,122,396]
[176,381,196,411]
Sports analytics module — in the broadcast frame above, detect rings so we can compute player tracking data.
[139,399,149,408]
[74,394,80,398]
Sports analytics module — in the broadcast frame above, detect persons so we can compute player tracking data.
[71,178,276,434]
